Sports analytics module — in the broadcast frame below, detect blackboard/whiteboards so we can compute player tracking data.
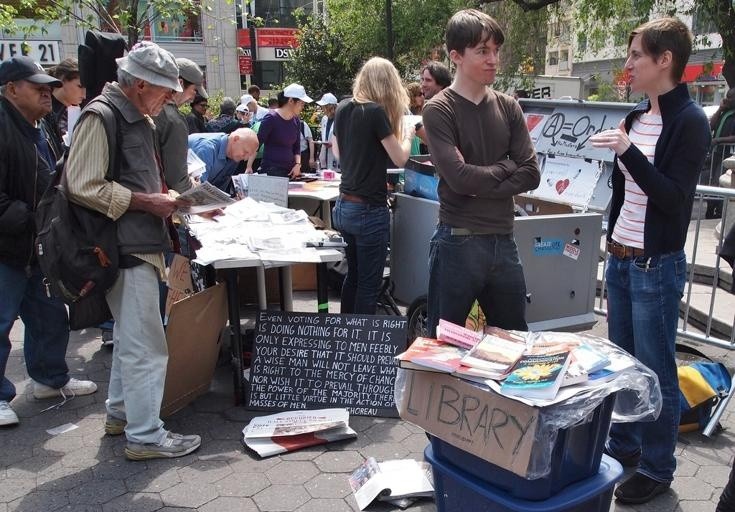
[245,310,410,418]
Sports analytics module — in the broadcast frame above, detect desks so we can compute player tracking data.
[166,168,361,406]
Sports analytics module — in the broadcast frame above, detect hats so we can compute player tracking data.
[114,40,209,106]
[0,55,64,89]
[220,94,254,115]
[283,83,314,104]
[315,92,338,106]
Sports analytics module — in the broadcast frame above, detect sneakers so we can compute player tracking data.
[105,414,127,436]
[614,472,671,504]
[124,430,202,461]
[100,329,113,343]
[33,377,98,400]
[0,399,21,428]
[603,443,642,468]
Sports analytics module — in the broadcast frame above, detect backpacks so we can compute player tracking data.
[33,98,124,305]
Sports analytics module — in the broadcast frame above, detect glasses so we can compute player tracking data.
[198,104,211,109]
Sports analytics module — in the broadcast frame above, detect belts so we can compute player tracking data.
[339,193,363,203]
[607,239,645,260]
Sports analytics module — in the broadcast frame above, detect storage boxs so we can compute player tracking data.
[422,371,619,501]
[425,442,624,511]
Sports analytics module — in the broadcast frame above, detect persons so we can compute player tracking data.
[37,56,87,156]
[186,59,451,200]
[331,55,416,313]
[420,6,543,338]
[151,57,209,194]
[589,16,713,505]
[0,56,98,428]
[63,39,225,462]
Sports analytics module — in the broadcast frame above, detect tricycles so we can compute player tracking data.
[377,97,638,349]
